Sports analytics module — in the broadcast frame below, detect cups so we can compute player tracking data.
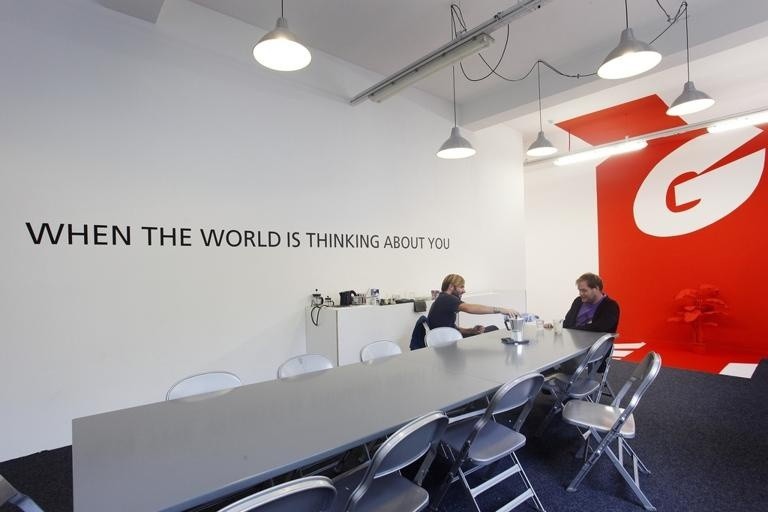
[352,296,362,304]
[536,320,545,332]
[553,320,563,335]
[365,296,377,305]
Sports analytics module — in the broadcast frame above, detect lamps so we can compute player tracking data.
[349,1,551,108]
[253,0,312,71]
[597,0,662,79]
[436,4,477,159]
[526,62,557,158]
[665,2,716,116]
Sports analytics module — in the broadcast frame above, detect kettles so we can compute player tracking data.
[339,290,356,306]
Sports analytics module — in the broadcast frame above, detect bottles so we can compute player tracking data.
[311,288,323,307]
[504,315,524,341]
[325,295,334,307]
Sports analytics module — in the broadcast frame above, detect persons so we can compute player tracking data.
[545,274,621,375]
[427,275,519,336]
